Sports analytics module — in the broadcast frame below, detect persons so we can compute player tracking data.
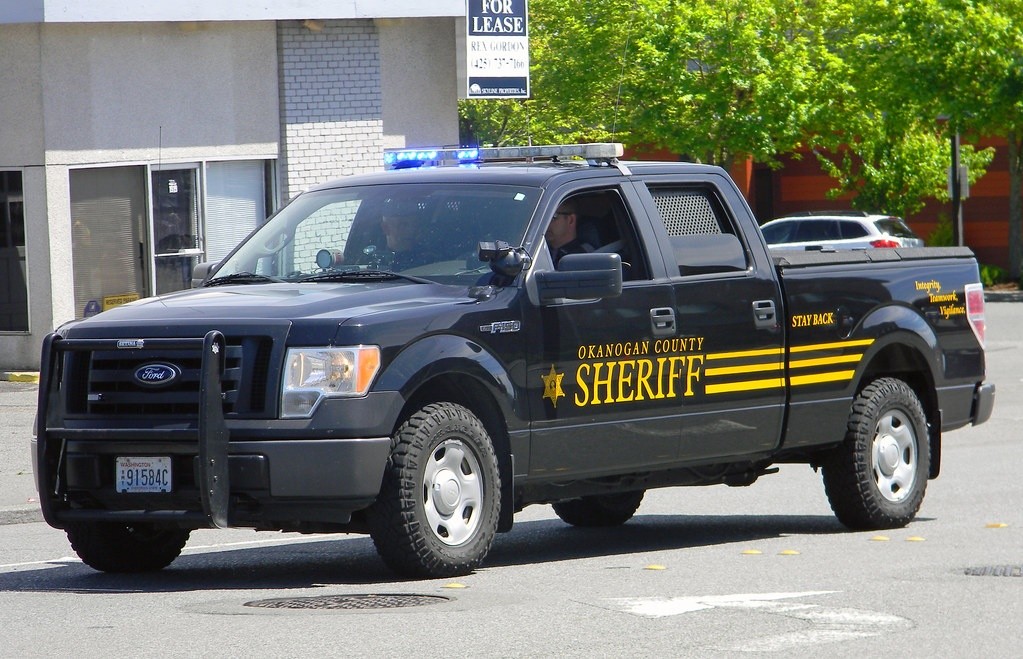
[354,197,438,273]
[544,198,595,271]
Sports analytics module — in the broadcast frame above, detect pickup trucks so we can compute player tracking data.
[31,146,996,580]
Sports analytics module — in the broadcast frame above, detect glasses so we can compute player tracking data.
[552,213,559,221]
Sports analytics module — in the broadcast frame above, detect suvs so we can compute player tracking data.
[757,209,924,256]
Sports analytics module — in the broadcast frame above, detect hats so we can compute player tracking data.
[557,198,580,214]
